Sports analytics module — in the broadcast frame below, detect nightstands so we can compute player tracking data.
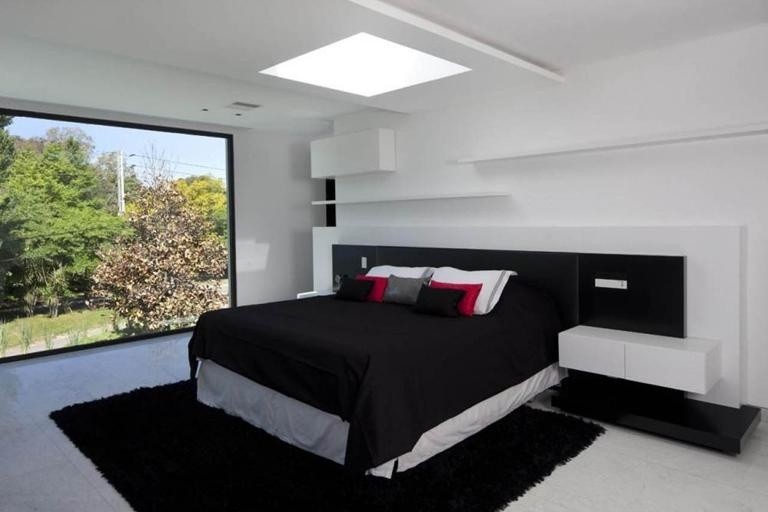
[556,324,723,397]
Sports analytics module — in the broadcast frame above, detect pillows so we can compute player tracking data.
[334,258,533,319]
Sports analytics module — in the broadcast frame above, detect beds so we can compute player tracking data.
[186,242,579,485]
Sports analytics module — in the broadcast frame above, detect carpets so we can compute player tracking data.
[47,375,607,511]
